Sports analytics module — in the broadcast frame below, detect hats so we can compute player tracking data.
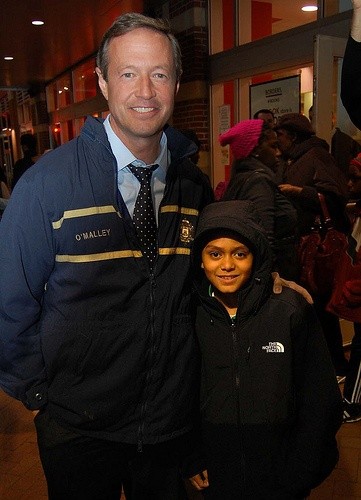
[273,113,315,134]
[220,120,264,160]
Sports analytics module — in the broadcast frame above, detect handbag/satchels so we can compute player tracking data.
[295,192,349,293]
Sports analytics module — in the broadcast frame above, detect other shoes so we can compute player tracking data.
[342,399,361,422]
[336,375,346,383]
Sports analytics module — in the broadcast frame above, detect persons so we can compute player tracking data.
[219,107,361,424]
[177,199,343,500]
[0,13,216,500]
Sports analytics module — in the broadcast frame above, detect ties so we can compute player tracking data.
[126,163,159,259]
